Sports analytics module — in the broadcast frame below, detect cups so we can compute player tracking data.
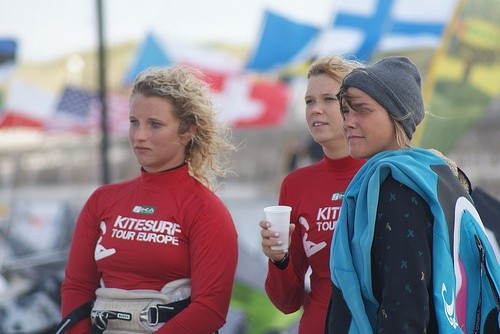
[264,206,292,251]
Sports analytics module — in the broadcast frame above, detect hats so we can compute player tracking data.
[342,57,425,140]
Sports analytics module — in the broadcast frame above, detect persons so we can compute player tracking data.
[259,55,367,334]
[61,63,239,334]
[323,56,500,334]
